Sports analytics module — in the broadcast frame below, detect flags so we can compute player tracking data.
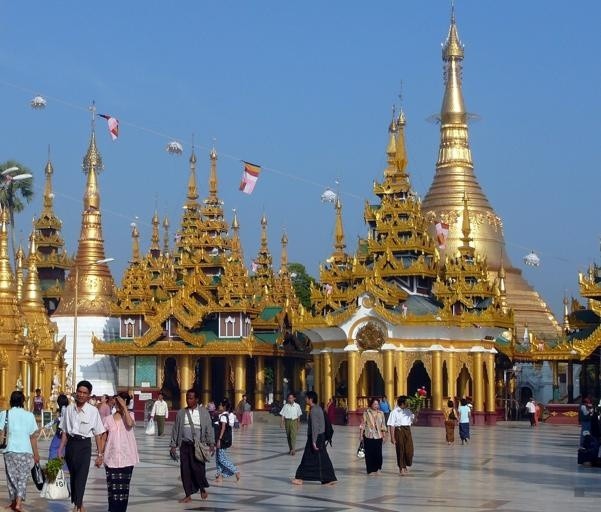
[99,113,120,142]
[172,233,409,317]
[433,221,451,250]
[239,161,263,195]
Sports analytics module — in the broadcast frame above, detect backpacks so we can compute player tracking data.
[323,407,333,446]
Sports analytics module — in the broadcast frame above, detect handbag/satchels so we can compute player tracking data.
[357,441,365,459]
[0,429,7,449]
[449,409,457,420]
[31,464,70,500]
[195,414,232,461]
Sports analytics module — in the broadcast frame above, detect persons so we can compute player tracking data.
[326,398,332,408]
[290,391,339,486]
[328,396,338,423]
[381,396,391,425]
[360,398,388,475]
[387,396,418,476]
[1,379,255,512]
[445,400,459,444]
[578,394,601,469]
[527,397,536,431]
[533,401,540,427]
[280,393,303,456]
[458,399,472,444]
